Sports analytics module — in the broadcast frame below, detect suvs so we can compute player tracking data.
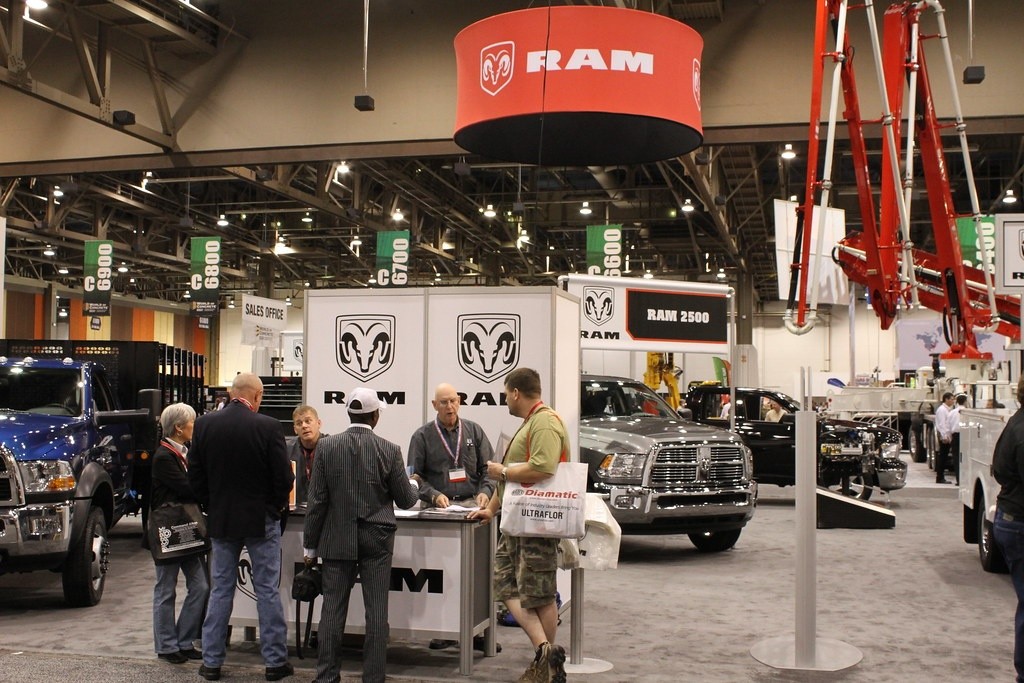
[581,374,758,551]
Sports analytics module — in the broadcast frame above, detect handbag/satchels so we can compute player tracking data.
[499,413,589,539]
[147,501,213,567]
[292,563,323,602]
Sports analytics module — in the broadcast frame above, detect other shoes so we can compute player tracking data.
[936,479,952,484]
[180,649,203,660]
[158,652,188,664]
[473,634,502,653]
[428,639,458,649]
[266,663,294,681]
[199,663,220,680]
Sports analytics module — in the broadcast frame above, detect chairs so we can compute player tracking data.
[35,374,77,408]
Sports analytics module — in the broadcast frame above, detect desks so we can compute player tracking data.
[227,504,502,676]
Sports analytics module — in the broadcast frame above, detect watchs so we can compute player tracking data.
[501,467,508,481]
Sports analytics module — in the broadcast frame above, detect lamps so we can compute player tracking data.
[351,236,362,245]
[580,202,591,214]
[483,205,496,217]
[393,208,403,220]
[781,144,796,159]
[1003,189,1017,203]
[302,212,312,222]
[682,199,694,211]
[217,214,228,226]
[519,230,529,241]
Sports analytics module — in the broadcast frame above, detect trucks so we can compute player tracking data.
[0,338,209,610]
[825,367,1019,473]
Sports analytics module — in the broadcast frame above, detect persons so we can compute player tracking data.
[677,399,685,410]
[992,376,1024,683]
[765,401,788,422]
[142,403,210,663]
[407,384,502,652]
[813,401,827,412]
[186,372,294,682]
[487,369,571,683]
[936,392,954,483]
[947,396,967,485]
[214,397,227,411]
[303,389,422,683]
[985,399,998,408]
[716,396,731,418]
[287,405,331,501]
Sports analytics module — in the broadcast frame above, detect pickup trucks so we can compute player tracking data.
[960,409,1013,574]
[683,382,909,500]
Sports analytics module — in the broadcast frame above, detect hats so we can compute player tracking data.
[345,388,386,414]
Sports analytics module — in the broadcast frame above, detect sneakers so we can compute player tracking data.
[514,643,567,683]
